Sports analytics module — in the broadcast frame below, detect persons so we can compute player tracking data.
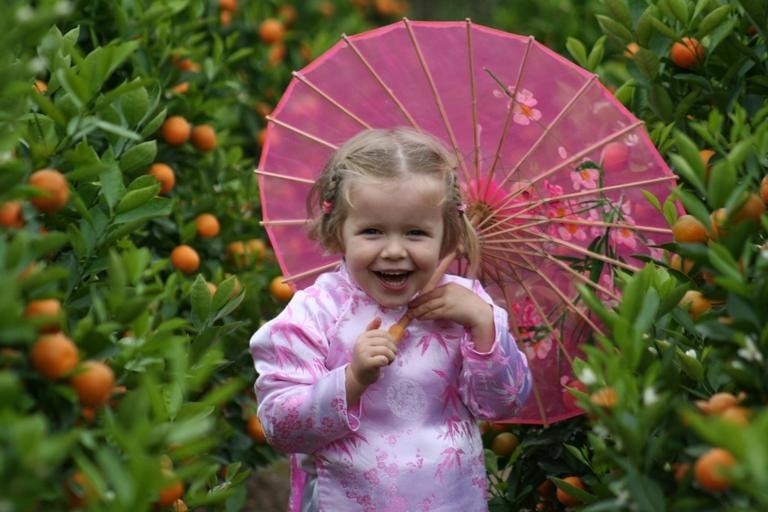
[243,124,532,508]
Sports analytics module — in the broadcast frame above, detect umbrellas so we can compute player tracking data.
[253,16,686,434]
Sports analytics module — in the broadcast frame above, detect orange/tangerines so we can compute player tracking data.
[0,0,768,512]
